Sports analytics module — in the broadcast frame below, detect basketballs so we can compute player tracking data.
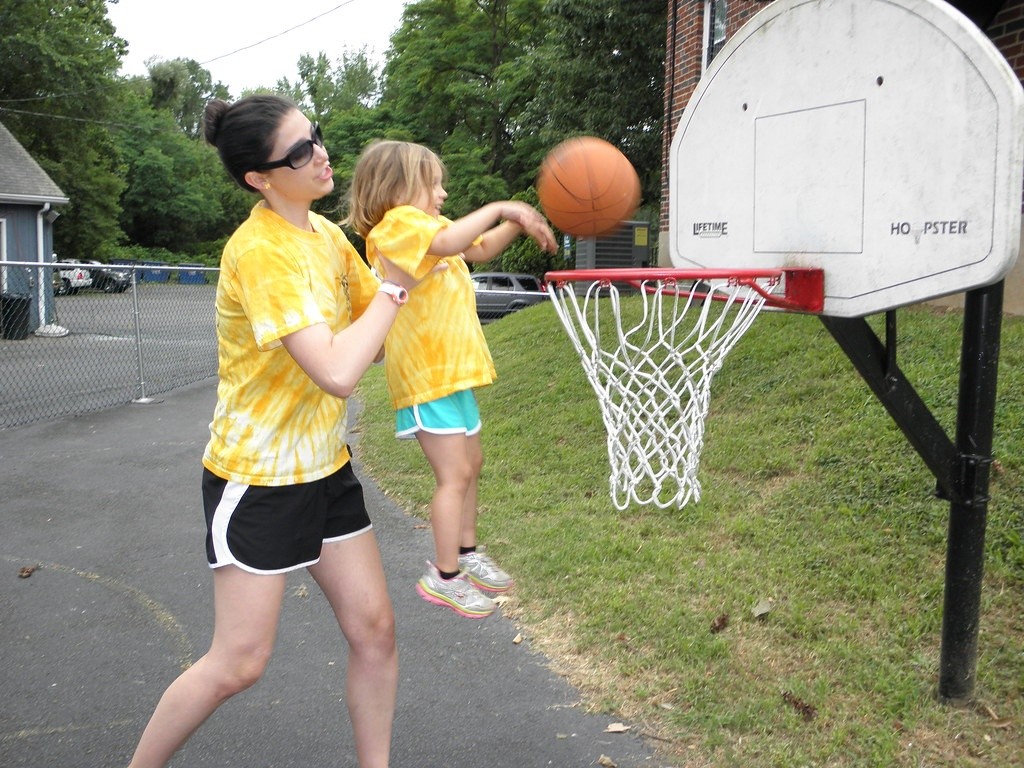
[536,136,639,237]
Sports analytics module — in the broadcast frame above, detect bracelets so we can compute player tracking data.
[386,278,408,290]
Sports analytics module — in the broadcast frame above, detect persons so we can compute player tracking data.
[126,97,448,767]
[343,141,561,619]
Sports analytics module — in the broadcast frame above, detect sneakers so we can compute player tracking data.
[416,561,496,618]
[457,545,512,591]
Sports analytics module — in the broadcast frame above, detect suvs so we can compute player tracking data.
[79,258,131,294]
[52,260,94,296]
[470,272,550,319]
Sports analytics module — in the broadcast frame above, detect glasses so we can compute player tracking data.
[254,120,323,170]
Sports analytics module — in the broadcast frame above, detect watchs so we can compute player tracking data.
[375,280,408,306]
